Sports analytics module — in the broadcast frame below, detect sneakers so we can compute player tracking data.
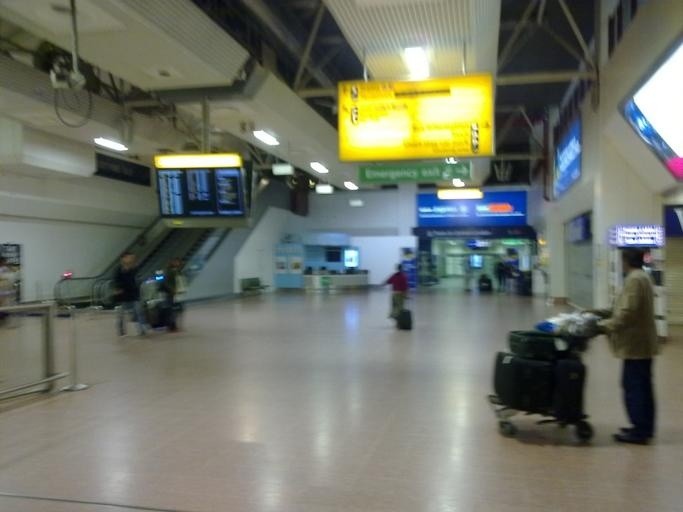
[611,432,651,445]
[618,424,653,437]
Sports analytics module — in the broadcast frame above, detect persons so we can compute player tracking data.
[384,264,409,319]
[112,252,147,337]
[498,261,514,293]
[163,257,183,330]
[581,248,659,444]
[464,259,473,291]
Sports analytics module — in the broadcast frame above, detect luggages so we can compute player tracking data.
[491,349,587,420]
[396,295,414,330]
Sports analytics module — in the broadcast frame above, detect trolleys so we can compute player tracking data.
[124,276,183,330]
[482,319,597,445]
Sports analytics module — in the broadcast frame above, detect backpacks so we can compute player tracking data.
[507,324,582,360]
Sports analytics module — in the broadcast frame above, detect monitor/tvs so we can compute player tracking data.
[151,152,253,229]
[617,28,683,185]
[343,248,359,268]
